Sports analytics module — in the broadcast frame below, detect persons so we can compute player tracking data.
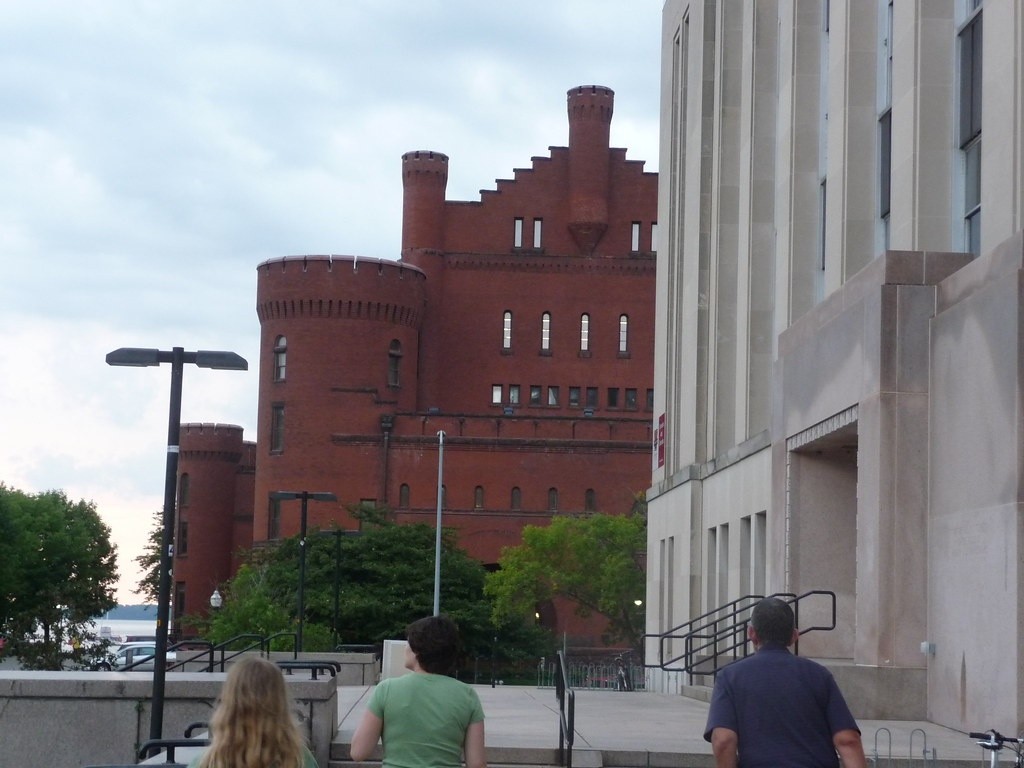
[349,616,486,768]
[187,658,318,768]
[704,600,869,768]
[0,635,7,663]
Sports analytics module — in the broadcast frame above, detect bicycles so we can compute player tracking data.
[614,649,634,689]
[967,728,1024,768]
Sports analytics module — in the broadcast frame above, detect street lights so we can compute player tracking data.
[105,345,249,756]
[269,491,337,652]
[432,431,447,618]
[318,529,361,650]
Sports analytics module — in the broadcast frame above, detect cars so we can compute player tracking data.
[90,645,177,675]
